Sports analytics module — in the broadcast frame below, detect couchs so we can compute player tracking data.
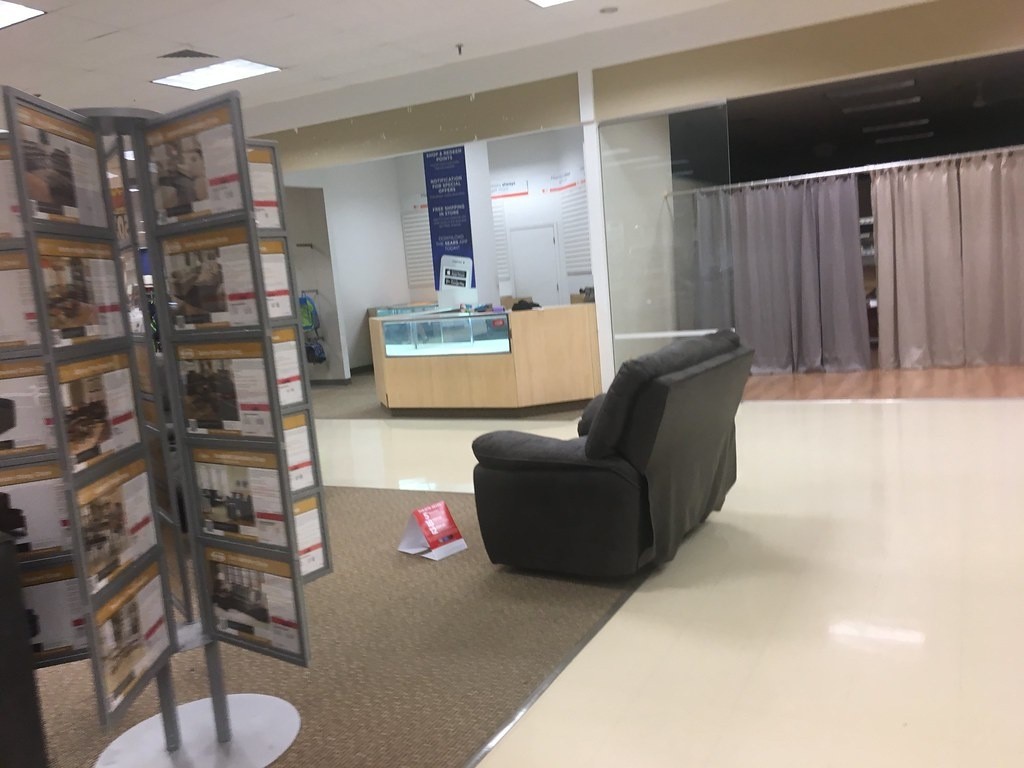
[470,330,754,582]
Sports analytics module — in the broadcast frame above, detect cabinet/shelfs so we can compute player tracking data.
[366,301,602,418]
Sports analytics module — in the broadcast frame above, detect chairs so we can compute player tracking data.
[570,293,585,303]
[500,296,532,309]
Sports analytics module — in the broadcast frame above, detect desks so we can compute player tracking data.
[387,302,436,342]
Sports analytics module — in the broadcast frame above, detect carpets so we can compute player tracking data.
[36,486,635,768]
